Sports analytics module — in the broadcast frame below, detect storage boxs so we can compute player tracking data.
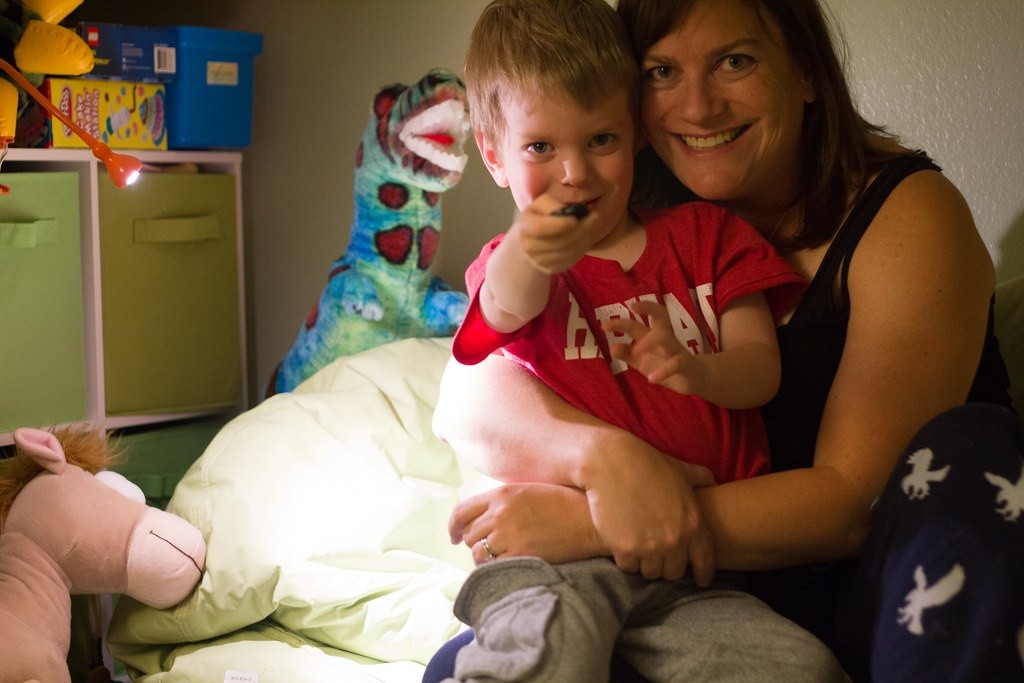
[7,77,167,151]
[78,22,264,150]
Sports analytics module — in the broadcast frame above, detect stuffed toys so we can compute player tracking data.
[271,72,472,397]
[0,424,206,683]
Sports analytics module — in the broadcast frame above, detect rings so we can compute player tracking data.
[482,537,497,559]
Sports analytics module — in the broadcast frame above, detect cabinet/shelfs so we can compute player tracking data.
[1,148,246,682]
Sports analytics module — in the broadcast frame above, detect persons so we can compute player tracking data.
[442,1,852,683]
[421,0,1024,683]
